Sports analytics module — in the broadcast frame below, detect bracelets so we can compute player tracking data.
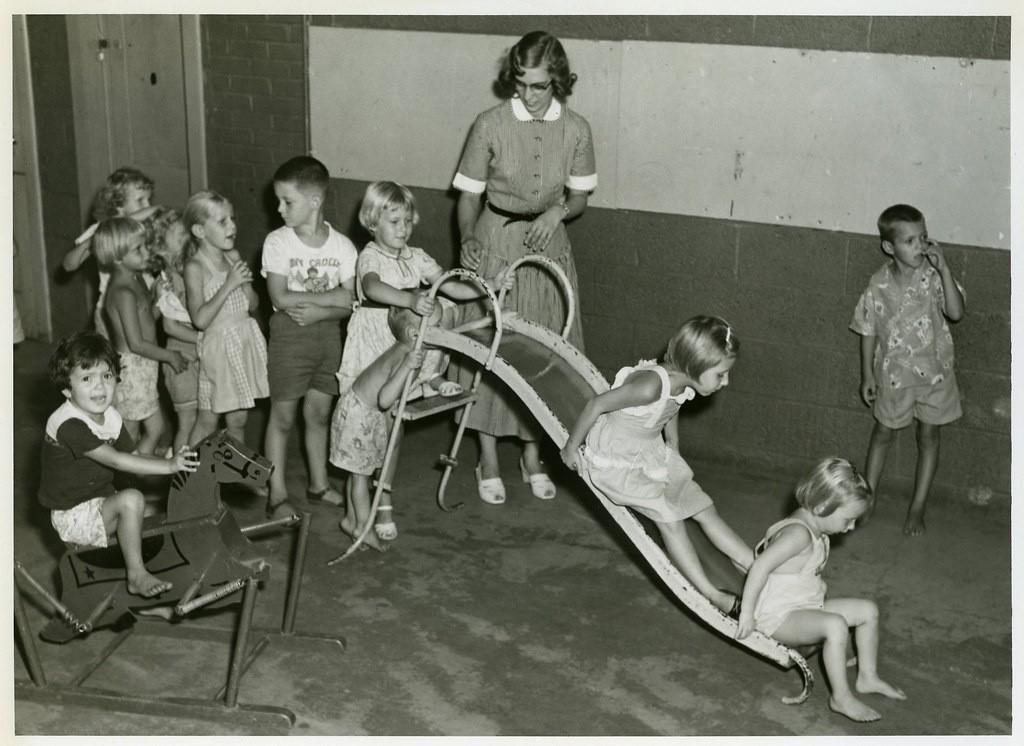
[558,201,570,217]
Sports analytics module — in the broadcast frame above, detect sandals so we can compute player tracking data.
[266,498,301,520]
[423,373,462,398]
[376,506,399,540]
[307,486,345,507]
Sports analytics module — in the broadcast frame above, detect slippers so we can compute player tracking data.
[718,588,741,621]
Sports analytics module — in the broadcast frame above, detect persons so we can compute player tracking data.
[559,313,757,620]
[448,30,599,507]
[849,204,967,538]
[36,330,200,597]
[62,166,267,458]
[734,456,906,722]
[328,289,454,554]
[260,158,358,530]
[338,178,514,541]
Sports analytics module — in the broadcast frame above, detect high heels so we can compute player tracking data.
[475,464,506,504]
[520,457,556,499]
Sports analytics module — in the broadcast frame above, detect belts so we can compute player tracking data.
[486,203,544,226]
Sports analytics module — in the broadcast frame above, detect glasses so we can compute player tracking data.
[515,78,553,95]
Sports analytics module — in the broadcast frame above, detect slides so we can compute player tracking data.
[423,307,825,671]
